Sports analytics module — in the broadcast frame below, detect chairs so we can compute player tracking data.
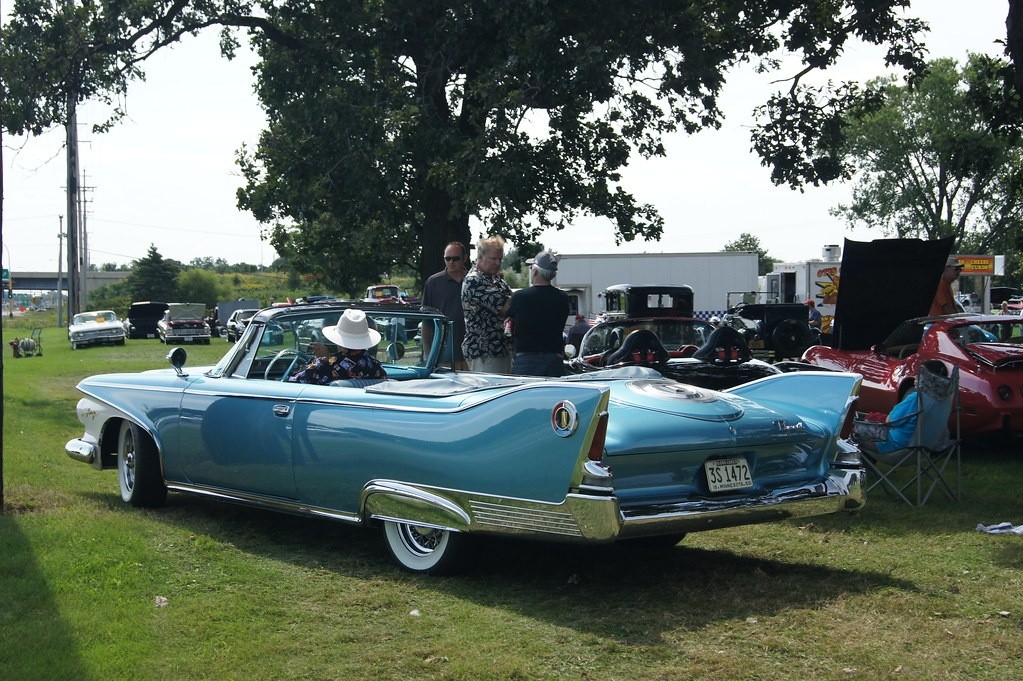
[332,379,397,388]
[960,329,989,344]
[607,329,671,366]
[697,327,754,360]
[849,365,963,509]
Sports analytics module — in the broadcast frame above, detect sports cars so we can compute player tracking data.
[800,236,1023,445]
[560,280,823,392]
[60,298,870,577]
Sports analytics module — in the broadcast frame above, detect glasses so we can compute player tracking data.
[444,256,463,261]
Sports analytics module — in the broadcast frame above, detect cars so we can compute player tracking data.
[212,284,424,348]
[68,310,127,350]
[155,302,212,346]
[124,301,168,339]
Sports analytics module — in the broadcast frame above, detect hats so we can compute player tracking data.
[946,257,964,267]
[805,300,814,306]
[525,252,557,271]
[322,309,381,349]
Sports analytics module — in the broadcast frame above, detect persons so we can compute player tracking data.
[511,250,570,376]
[710,314,720,321]
[421,242,470,371]
[462,236,514,375]
[930,257,965,314]
[287,309,387,386]
[999,301,1013,315]
[567,315,591,356]
[804,299,821,329]
